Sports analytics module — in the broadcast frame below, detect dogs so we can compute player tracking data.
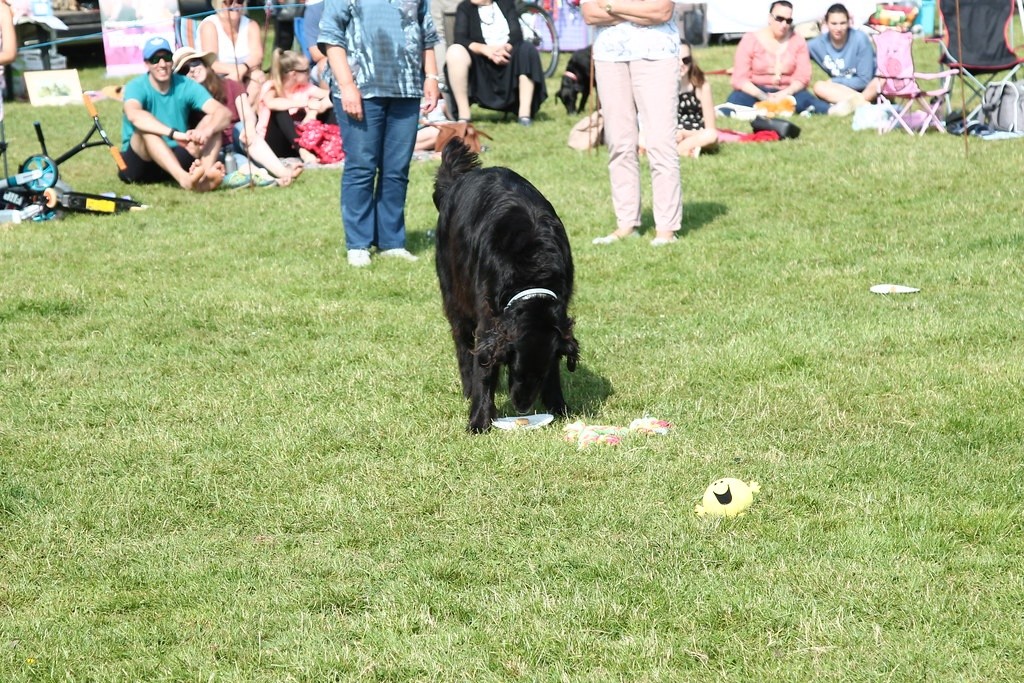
[432,133,582,435]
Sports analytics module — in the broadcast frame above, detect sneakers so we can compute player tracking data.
[347,249,371,267]
[380,248,417,260]
[247,169,276,188]
[218,171,252,191]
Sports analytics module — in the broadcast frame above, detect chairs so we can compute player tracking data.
[869,30,958,135]
[924,0,1024,115]
[174,17,202,49]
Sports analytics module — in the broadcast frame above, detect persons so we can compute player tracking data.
[580,0,683,245]
[119,0,440,193]
[0,3,17,65]
[317,0,441,267]
[443,0,549,126]
[806,3,893,116]
[722,0,833,115]
[636,40,718,158]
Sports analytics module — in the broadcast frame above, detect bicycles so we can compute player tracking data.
[0,94,141,220]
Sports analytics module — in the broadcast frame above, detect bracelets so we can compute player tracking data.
[242,62,250,73]
[167,127,178,140]
[605,4,611,14]
[426,74,439,81]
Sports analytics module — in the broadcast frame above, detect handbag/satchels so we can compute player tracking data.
[427,122,494,154]
[977,81,1024,133]
[750,116,800,139]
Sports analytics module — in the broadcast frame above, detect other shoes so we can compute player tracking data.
[518,116,532,125]
[650,236,679,247]
[827,98,851,116]
[593,233,637,244]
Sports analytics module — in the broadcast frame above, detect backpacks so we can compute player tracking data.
[294,120,347,164]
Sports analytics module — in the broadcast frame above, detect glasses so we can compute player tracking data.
[680,55,692,65]
[286,69,309,74]
[148,54,172,65]
[771,11,793,25]
[223,0,245,6]
[178,59,203,76]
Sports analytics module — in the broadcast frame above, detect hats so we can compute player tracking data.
[172,46,217,73]
[143,37,174,62]
